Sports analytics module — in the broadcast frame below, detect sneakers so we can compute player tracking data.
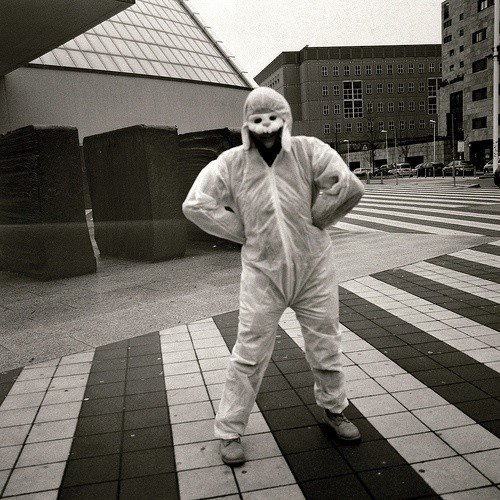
[219,437,247,465]
[321,407,361,440]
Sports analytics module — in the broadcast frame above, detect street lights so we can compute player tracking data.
[343,140,349,168]
[430,120,436,162]
[381,130,388,162]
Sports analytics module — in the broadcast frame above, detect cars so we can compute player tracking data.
[483,156,500,174]
[416,162,445,177]
[353,162,418,177]
[441,160,474,176]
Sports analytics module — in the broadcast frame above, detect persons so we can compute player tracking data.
[183,88,365,468]
